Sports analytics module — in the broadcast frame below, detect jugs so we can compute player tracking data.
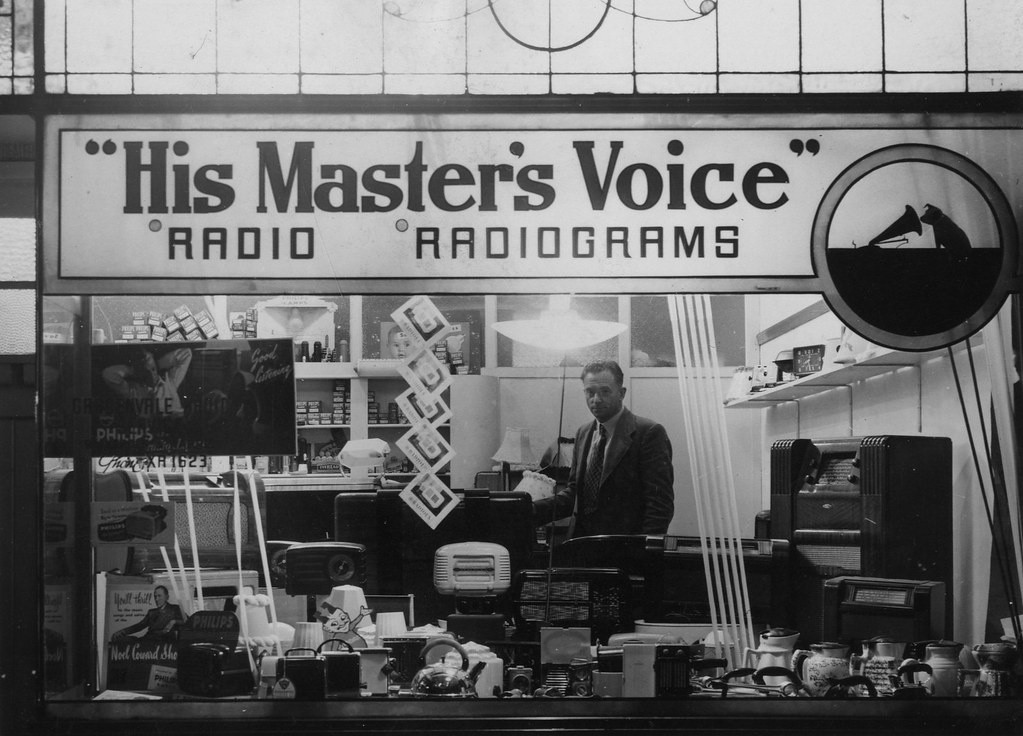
[739,627,1017,697]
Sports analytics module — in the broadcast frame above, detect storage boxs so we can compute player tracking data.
[115,305,219,344]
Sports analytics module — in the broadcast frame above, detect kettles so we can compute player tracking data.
[380,637,486,696]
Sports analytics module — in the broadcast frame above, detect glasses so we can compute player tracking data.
[582,387,620,398]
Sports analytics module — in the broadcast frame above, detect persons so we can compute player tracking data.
[112,586,186,651]
[532,359,674,646]
[386,325,411,360]
[102,348,193,453]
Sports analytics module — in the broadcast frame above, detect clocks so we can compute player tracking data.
[792,344,825,376]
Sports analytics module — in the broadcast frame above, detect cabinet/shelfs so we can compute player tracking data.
[217,294,500,491]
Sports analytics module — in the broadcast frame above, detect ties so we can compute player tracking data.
[579,423,609,526]
